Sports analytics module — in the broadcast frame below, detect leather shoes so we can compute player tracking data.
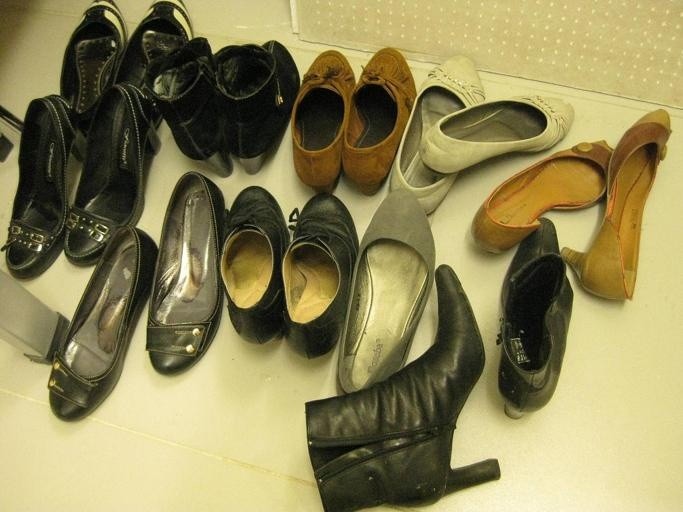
[280,191,359,362]
[221,186,290,348]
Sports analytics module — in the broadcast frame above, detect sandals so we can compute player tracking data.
[335,189,436,396]
[58,0,128,164]
[113,0,193,131]
[418,94,574,175]
[45,225,156,425]
[144,171,226,378]
[388,54,486,215]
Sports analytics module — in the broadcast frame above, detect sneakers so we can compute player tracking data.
[290,50,356,192]
[342,47,417,197]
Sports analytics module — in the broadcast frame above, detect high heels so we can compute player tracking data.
[471,138,615,254]
[62,84,160,266]
[211,39,300,176]
[560,108,672,299]
[4,95,79,281]
[497,217,574,421]
[305,263,500,511]
[144,36,234,178]
